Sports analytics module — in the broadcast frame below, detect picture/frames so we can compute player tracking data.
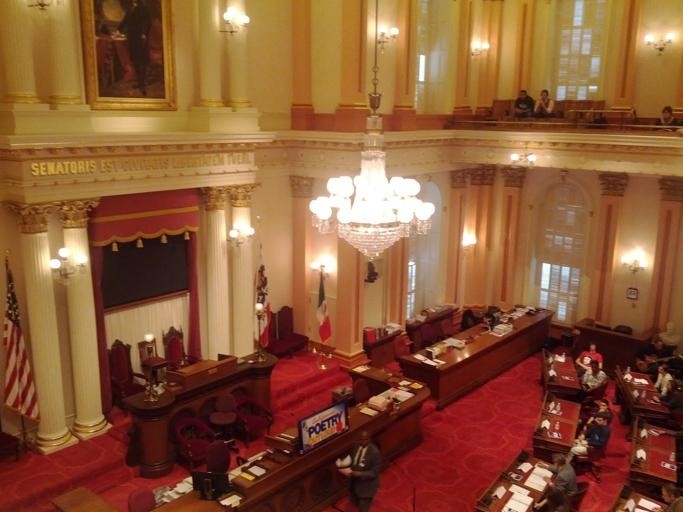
[625,287,638,300]
[78,0,179,111]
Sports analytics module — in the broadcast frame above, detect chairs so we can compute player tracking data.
[105,338,147,415]
[351,377,370,404]
[173,391,272,473]
[264,311,293,356]
[128,485,156,511]
[391,308,475,361]
[162,325,199,371]
[279,306,309,351]
[483,96,636,127]
[138,337,169,381]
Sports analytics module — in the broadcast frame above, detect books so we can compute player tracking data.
[359,394,388,417]
[491,303,536,337]
[152,476,192,507]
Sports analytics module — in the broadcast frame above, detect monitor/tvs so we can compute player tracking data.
[193,472,228,500]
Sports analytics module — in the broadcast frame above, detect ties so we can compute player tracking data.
[356,447,363,465]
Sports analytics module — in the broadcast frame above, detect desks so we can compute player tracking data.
[401,303,555,410]
[474,317,680,512]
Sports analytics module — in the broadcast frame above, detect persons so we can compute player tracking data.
[500,340,683,511]
[513,89,533,118]
[337,429,379,511]
[0,255,39,445]
[653,106,676,132]
[113,0,152,97]
[657,321,680,346]
[534,89,556,118]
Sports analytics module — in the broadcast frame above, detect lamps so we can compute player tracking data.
[47,246,89,279]
[376,27,399,51]
[509,147,537,167]
[619,249,651,274]
[645,33,675,54]
[222,6,250,30]
[229,219,256,246]
[309,0,435,262]
[310,252,338,279]
[469,41,489,56]
[254,302,266,362]
